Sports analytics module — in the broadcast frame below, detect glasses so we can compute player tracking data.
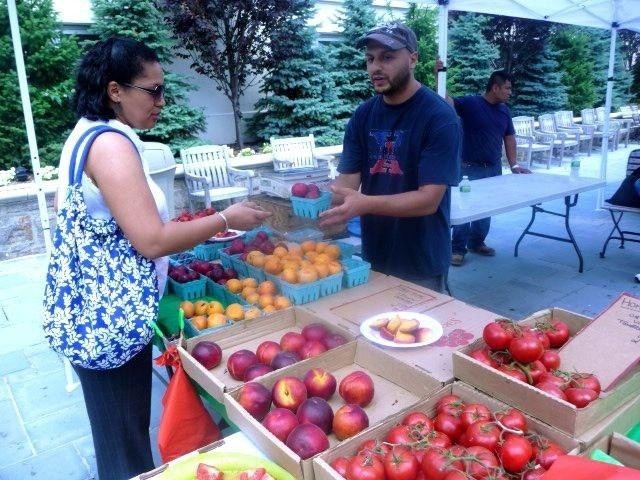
[123,82,163,101]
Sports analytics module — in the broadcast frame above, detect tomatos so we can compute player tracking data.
[332,392,567,480]
[468,315,600,410]
[170,207,239,238]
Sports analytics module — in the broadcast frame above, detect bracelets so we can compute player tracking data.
[511,165,520,169]
[215,211,230,234]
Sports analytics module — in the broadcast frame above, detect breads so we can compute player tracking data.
[367,314,432,343]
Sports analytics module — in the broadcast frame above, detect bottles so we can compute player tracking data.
[458,175,473,211]
[570,152,580,186]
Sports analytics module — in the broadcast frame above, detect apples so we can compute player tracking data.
[225,232,287,258]
[237,367,375,457]
[182,298,241,330]
[191,260,236,286]
[227,321,349,382]
[247,240,341,281]
[226,276,289,318]
[291,183,321,199]
[192,340,222,369]
[169,263,201,283]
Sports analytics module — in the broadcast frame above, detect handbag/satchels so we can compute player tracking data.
[43,126,159,369]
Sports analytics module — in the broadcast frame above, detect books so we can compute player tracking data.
[258,167,337,201]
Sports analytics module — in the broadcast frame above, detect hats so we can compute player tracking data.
[356,22,416,54]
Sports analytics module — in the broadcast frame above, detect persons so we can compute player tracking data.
[433,58,534,266]
[42,39,273,479]
[318,24,463,299]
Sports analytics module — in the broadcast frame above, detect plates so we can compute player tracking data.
[360,310,444,350]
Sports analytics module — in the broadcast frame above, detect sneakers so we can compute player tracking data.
[467,245,494,256]
[451,255,464,265]
